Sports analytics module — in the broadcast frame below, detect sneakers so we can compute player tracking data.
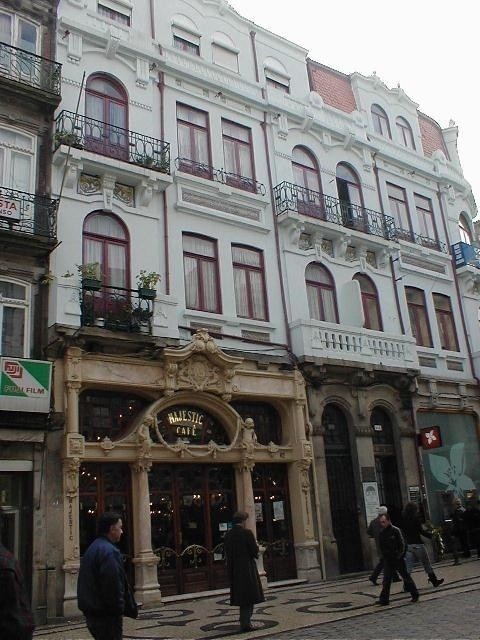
[375,600,391,605]
[368,575,380,587]
[393,578,403,582]
[410,595,420,602]
[242,623,261,631]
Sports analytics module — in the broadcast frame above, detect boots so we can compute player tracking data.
[427,576,445,588]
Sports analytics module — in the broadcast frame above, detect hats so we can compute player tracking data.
[378,505,388,515]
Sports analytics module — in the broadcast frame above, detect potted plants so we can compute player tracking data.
[54,129,155,168]
[77,262,159,334]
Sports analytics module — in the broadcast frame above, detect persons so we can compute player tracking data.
[0,541,36,640]
[375,515,420,606]
[240,416,258,460]
[77,511,128,640]
[223,512,267,632]
[138,414,155,460]
[364,506,402,586]
[401,502,446,592]
[443,496,480,568]
